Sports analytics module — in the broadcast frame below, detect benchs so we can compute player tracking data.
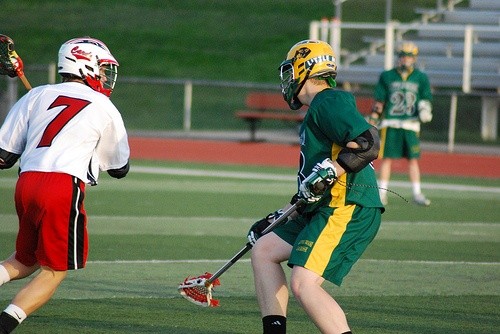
[233,93,375,143]
[336,0,500,90]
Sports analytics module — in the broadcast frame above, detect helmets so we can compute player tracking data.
[276,38,339,111]
[397,40,419,57]
[57,36,120,98]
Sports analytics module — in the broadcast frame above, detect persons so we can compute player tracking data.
[365,42,434,206]
[247,39,385,334]
[0,37,130,334]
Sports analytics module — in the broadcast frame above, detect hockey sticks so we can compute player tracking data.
[177,182,325,309]
[0,34,32,92]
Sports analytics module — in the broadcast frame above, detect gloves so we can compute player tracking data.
[297,158,338,206]
[247,209,288,247]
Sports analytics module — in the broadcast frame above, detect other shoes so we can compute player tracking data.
[413,194,431,208]
[380,194,389,208]
